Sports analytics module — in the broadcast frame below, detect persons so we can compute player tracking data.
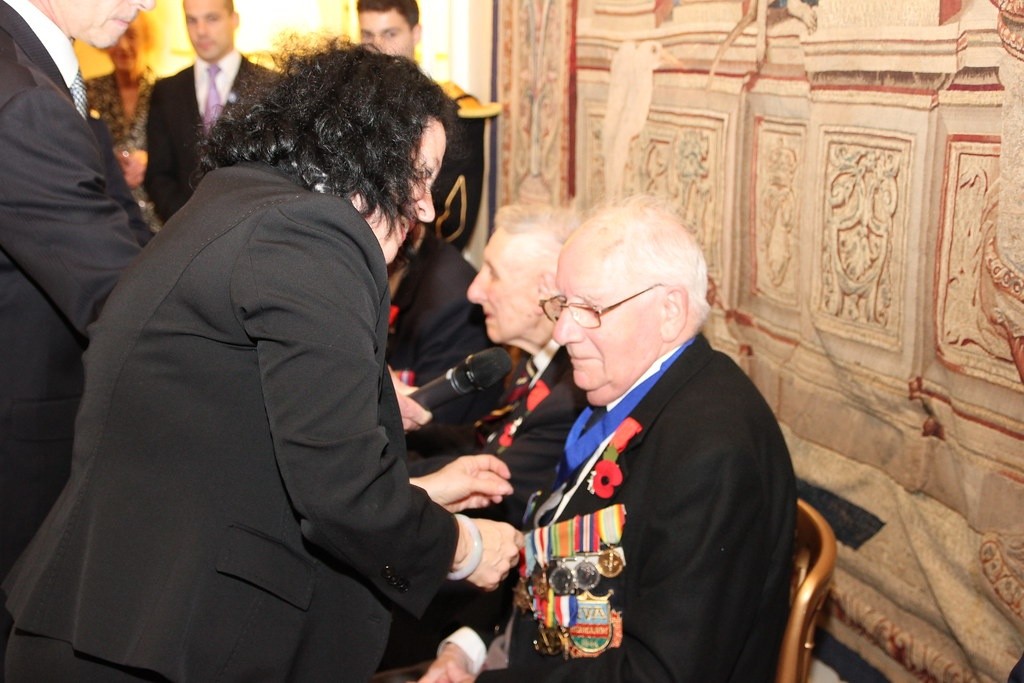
[0,0,525,683]
[419,194,798,683]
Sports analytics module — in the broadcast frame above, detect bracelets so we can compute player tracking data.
[446,514,483,581]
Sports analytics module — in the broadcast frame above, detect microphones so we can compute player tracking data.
[407,347,513,411]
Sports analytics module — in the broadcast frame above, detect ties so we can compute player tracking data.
[203,64,222,140]
[69,70,88,121]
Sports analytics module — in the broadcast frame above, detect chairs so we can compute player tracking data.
[773,496,838,683]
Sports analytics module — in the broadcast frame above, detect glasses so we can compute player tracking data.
[540,283,667,329]
